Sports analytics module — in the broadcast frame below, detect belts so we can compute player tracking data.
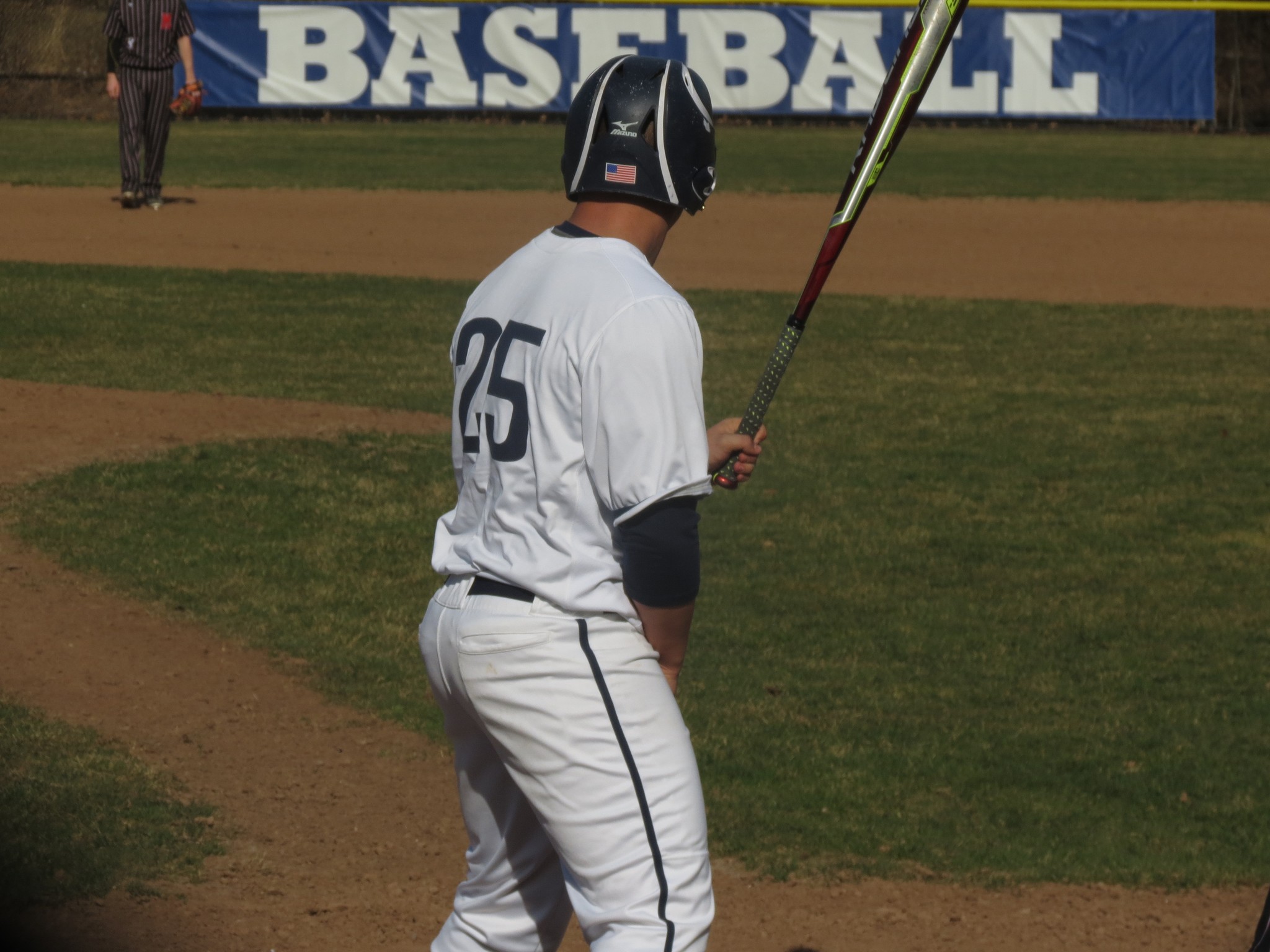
[468,575,534,602]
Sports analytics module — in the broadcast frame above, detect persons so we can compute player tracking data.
[103,0,203,211]
[417,55,767,952]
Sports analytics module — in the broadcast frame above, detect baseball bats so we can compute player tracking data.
[711,0,969,492]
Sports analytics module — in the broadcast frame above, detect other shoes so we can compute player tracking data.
[121,192,137,206]
[145,196,158,205]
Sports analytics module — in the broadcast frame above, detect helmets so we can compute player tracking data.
[562,51,719,214]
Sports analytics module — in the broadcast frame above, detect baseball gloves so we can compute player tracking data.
[169,80,203,117]
